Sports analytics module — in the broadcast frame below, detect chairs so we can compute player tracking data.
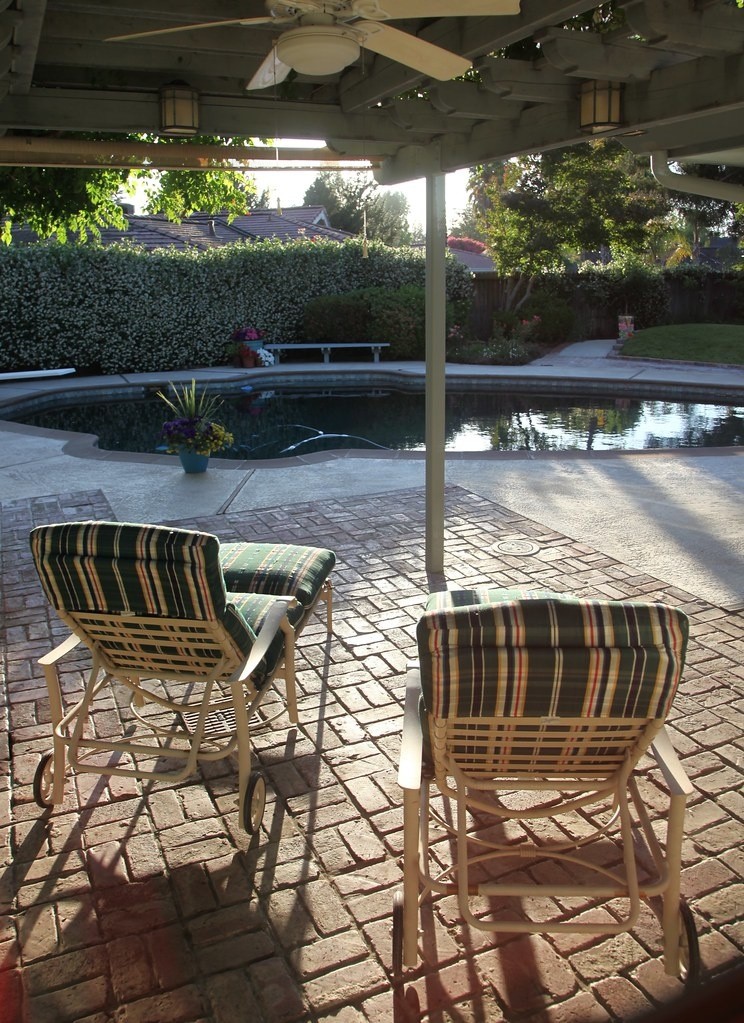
[28,520,338,836]
[389,589,701,989]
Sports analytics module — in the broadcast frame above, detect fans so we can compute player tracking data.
[104,0,521,91]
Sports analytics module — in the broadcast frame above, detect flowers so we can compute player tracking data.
[162,416,234,455]
[231,326,267,340]
[257,347,274,365]
[241,346,256,360]
[241,391,275,415]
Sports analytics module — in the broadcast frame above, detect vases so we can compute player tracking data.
[172,443,210,474]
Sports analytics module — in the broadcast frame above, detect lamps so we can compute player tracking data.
[579,79,627,135]
[158,79,201,134]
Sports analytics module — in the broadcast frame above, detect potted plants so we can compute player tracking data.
[227,342,246,368]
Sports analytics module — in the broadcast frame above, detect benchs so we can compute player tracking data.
[272,390,391,399]
[264,342,391,366]
